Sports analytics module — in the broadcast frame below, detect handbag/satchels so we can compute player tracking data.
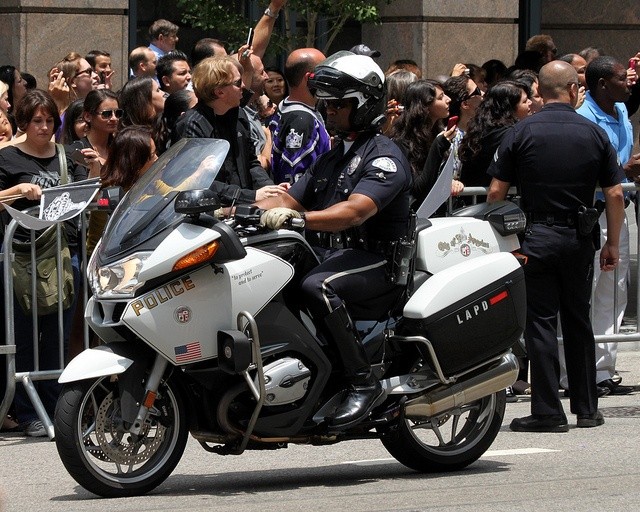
[13,221,76,317]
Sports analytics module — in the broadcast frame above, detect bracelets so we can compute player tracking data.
[263,7,279,20]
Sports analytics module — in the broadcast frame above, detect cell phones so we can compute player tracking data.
[247,28,254,49]
[448,116,458,136]
[629,59,636,82]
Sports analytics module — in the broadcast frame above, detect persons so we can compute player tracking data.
[118,76,167,125]
[192,38,229,66]
[0,65,70,138]
[459,81,586,404]
[130,18,180,89]
[509,79,545,116]
[559,53,640,118]
[384,59,422,82]
[61,98,89,143]
[66,89,123,369]
[487,60,625,436]
[86,125,164,346]
[212,53,419,432]
[511,69,540,88]
[382,69,418,105]
[48,52,99,143]
[272,48,331,186]
[393,80,464,217]
[128,47,159,77]
[440,75,485,198]
[230,0,287,170]
[0,81,11,115]
[155,45,255,146]
[261,66,288,105]
[85,49,115,89]
[525,35,557,64]
[188,55,293,206]
[557,56,640,398]
[156,52,191,99]
[451,64,488,93]
[256,93,278,119]
[0,89,101,436]
[515,51,546,75]
[580,47,640,87]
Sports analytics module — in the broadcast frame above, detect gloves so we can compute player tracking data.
[259,207,301,230]
[212,207,224,219]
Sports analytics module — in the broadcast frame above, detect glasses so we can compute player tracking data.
[74,68,93,74]
[464,86,481,101]
[323,99,347,110]
[233,79,242,87]
[551,48,558,55]
[74,117,85,123]
[94,109,124,119]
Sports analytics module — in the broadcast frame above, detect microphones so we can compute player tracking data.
[325,114,386,135]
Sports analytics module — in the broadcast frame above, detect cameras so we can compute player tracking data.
[462,68,470,75]
[390,105,406,114]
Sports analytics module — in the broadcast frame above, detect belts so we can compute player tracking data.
[325,235,397,253]
[532,213,579,226]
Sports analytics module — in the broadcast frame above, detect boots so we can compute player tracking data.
[327,299,387,432]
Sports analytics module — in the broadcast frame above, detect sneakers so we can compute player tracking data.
[506,387,518,403]
[22,419,47,436]
[1,415,21,431]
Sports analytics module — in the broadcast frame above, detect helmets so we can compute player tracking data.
[305,53,388,133]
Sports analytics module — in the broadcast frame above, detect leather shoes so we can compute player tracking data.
[577,409,604,427]
[597,385,611,398]
[597,378,633,395]
[510,414,569,432]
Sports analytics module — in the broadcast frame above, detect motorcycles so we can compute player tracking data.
[53,139,526,498]
